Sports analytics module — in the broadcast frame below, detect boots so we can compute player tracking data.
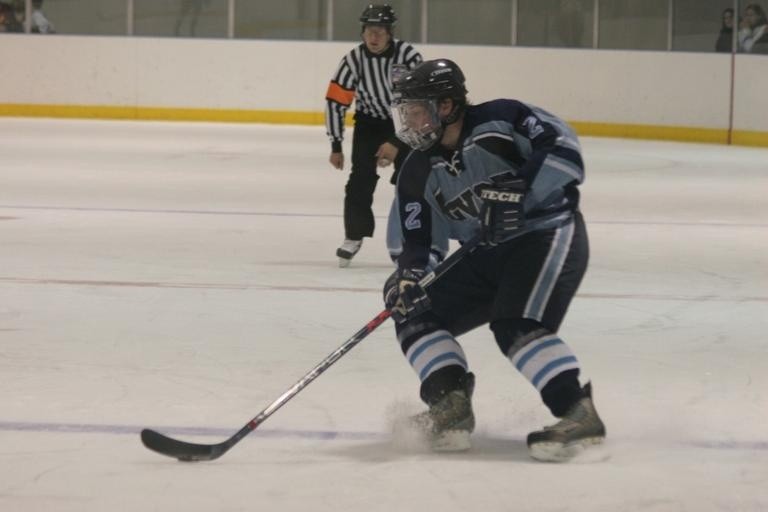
[406,372,475,433]
[526,378,607,449]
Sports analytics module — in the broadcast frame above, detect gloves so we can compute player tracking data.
[383,277,433,324]
[476,199,525,246]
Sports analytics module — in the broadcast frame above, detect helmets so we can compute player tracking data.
[358,3,398,25]
[390,58,469,104]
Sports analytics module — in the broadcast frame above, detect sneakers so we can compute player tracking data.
[336,238,363,259]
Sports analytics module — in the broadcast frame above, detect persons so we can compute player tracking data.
[0,0,55,34]
[716,5,768,54]
[323,4,423,272]
[382,59,606,462]
